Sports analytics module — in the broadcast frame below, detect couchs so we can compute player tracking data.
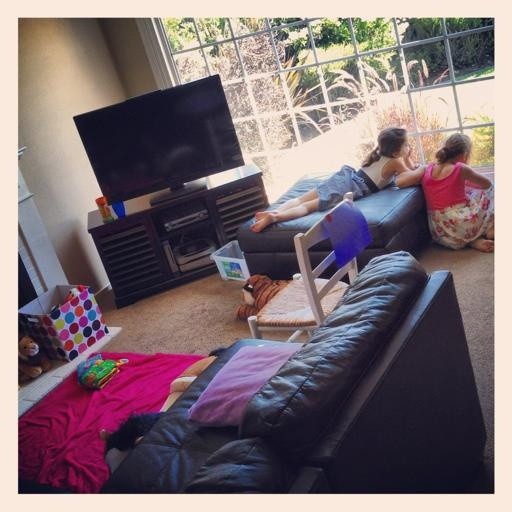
[99,250,488,494]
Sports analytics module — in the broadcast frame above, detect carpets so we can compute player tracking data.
[18,353,205,494]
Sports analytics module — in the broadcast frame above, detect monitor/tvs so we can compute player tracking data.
[72,73,245,206]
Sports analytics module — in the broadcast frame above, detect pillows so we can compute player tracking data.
[186,345,298,427]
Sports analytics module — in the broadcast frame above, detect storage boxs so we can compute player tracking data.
[18,285,110,361]
[210,240,250,282]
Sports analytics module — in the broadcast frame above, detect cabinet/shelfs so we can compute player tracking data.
[88,156,270,309]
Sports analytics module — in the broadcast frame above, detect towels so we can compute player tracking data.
[322,205,373,270]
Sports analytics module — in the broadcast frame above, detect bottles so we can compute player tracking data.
[94,196,113,223]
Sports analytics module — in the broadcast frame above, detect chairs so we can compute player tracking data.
[246,191,360,343]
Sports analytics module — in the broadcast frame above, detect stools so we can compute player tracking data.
[237,176,432,283]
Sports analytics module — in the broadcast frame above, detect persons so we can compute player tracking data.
[394,132,493,254]
[249,128,415,232]
[236,273,291,320]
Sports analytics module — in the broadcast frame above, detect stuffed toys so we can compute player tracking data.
[77,355,129,390]
[19,333,53,386]
